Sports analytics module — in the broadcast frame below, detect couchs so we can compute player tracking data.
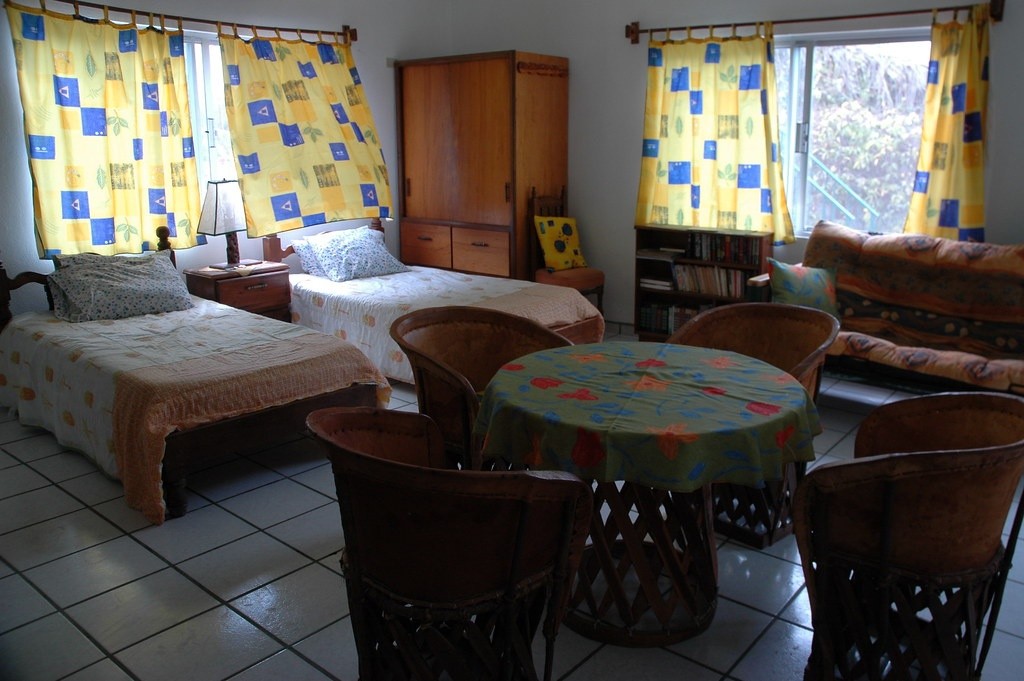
[746,220,1024,400]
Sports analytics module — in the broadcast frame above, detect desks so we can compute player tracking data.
[470,339,824,649]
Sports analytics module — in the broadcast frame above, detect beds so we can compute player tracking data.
[0,224,395,526]
[263,217,605,384]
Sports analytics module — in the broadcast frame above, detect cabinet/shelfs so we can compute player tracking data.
[634,223,775,343]
[393,50,569,283]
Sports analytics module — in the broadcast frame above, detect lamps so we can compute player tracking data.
[197,177,263,269]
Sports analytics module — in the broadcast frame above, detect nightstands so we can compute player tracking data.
[183,259,293,322]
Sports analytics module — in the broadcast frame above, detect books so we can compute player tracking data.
[639,301,698,336]
[636,232,760,265]
[636,263,757,299]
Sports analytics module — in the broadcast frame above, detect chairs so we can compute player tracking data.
[390,305,577,471]
[791,391,1024,681]
[664,301,840,549]
[530,184,606,318]
[305,405,594,681]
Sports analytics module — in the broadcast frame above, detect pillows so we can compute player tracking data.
[46,248,195,322]
[534,214,588,273]
[290,223,413,283]
[764,256,842,323]
